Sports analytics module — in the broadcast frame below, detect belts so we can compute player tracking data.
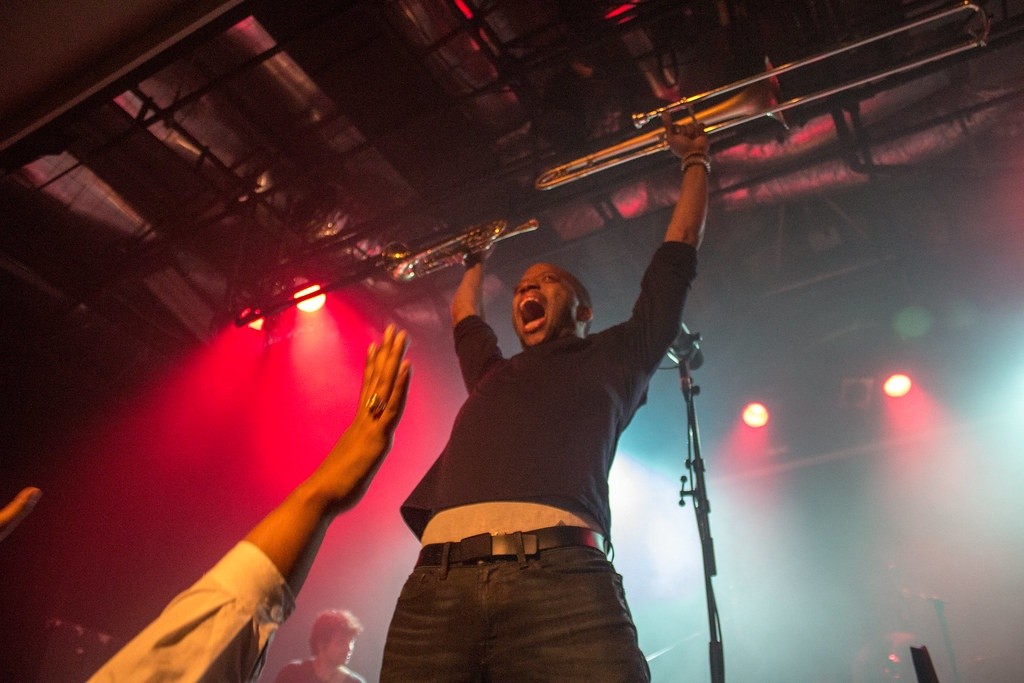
[416,526,608,568]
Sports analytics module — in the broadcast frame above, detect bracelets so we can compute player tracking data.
[680,151,712,173]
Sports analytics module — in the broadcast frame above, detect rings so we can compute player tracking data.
[365,393,386,416]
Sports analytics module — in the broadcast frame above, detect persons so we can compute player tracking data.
[272,609,365,683]
[379,121,712,683]
[85,324,411,683]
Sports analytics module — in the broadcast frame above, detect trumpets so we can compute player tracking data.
[391,217,540,284]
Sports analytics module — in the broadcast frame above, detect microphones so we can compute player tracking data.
[668,324,704,371]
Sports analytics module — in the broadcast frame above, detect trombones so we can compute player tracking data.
[533,3,994,195]
